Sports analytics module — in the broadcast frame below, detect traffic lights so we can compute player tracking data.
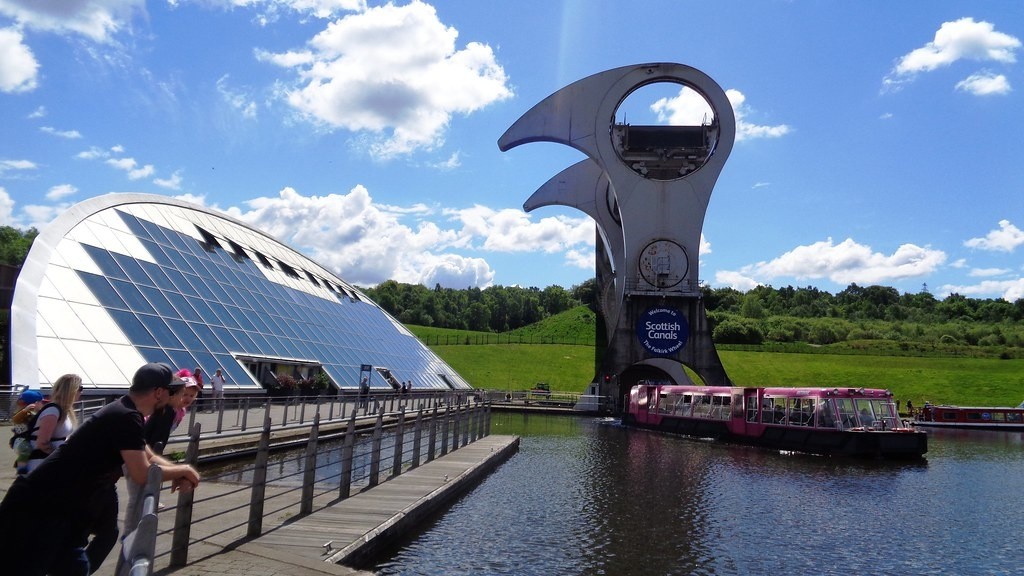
[605,374,611,383]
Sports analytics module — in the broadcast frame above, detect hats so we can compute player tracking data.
[179,375,202,392]
[18,390,52,405]
[134,362,173,385]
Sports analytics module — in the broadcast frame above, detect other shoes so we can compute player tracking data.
[157,502,165,509]
[119,535,127,543]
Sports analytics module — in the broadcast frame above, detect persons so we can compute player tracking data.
[211,369,225,413]
[13,389,50,477]
[896,399,900,410]
[194,367,203,412]
[144,369,200,431]
[120,377,183,544]
[907,400,912,411]
[860,409,874,426]
[29,375,82,473]
[0,363,199,576]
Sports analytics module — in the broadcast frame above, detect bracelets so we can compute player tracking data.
[221,375,222,377]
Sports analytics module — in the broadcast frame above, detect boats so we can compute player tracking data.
[909,405,1024,430]
[621,385,928,462]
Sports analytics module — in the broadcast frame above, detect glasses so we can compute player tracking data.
[78,386,83,392]
[154,385,175,397]
